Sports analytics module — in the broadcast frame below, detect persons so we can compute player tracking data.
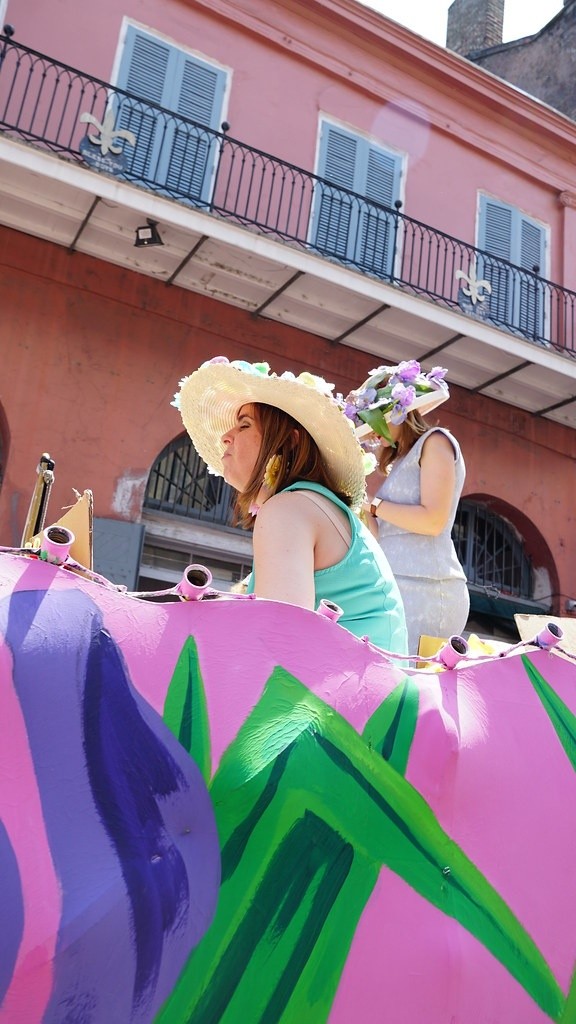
[174,355,408,665]
[344,359,472,670]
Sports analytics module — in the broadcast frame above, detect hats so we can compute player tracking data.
[343,360,450,443]
[171,356,376,515]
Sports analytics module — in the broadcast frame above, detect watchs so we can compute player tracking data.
[370,496,384,518]
